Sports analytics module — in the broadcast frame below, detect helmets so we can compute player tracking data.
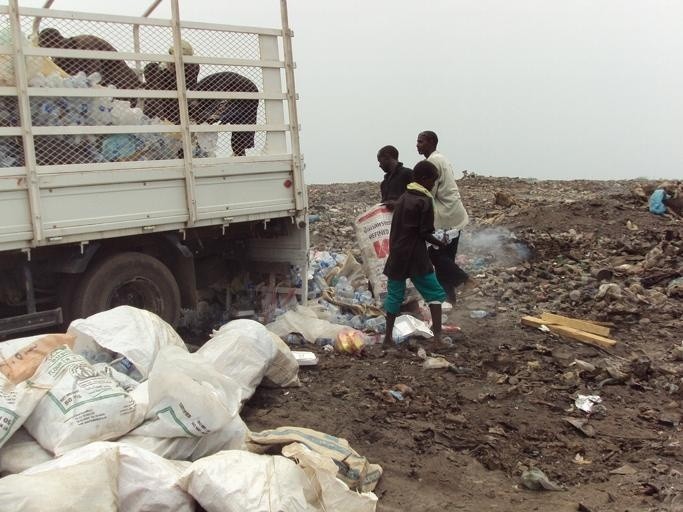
[168,40,193,55]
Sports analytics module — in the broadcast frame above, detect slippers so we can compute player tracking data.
[456,288,480,299]
[436,344,457,352]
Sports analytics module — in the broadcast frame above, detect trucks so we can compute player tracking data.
[0,0,310,340]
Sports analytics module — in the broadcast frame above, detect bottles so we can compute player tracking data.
[365,315,387,329]
[469,310,488,318]
[372,323,384,334]
[285,249,373,330]
[9,70,178,160]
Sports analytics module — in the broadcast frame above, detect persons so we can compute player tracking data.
[646,187,682,222]
[175,71,258,155]
[382,160,449,349]
[366,145,415,300]
[140,41,200,123]
[39,28,139,109]
[386,133,477,307]
